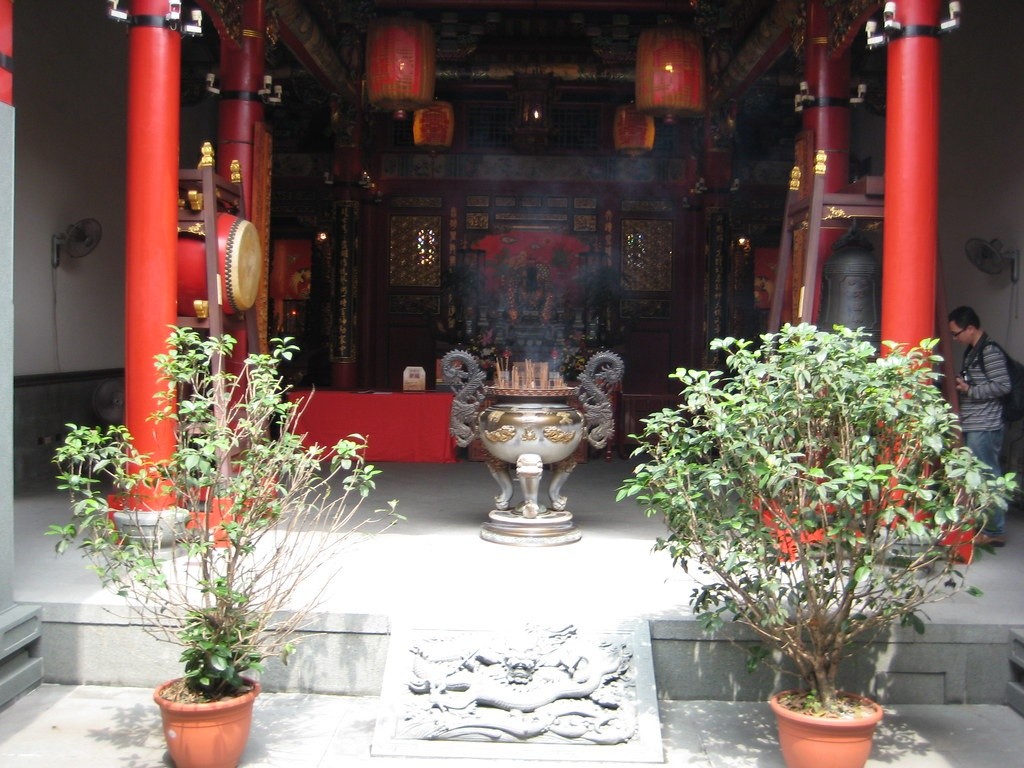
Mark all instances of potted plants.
[616,323,1018,768]
[43,325,408,767]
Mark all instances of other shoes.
[974,533,1007,546]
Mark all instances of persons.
[948,306,1011,547]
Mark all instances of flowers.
[465,328,512,379]
[548,331,598,381]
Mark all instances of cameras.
[956,370,968,384]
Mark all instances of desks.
[285,390,457,462]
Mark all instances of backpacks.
[978,341,1024,422]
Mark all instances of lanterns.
[413,101,454,153]
[365,13,436,121]
[636,16,706,126]
[613,104,655,162]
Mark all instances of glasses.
[951,329,966,336]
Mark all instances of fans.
[52,218,102,266]
[964,236,1019,282]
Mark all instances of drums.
[176,210,263,317]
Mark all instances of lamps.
[504,60,566,156]
[268,85,282,103]
[205,73,220,94]
[865,20,884,46]
[323,170,384,203]
[883,2,900,30]
[800,80,815,101]
[848,84,867,103]
[165,0,181,21]
[682,177,740,207]
[108,0,126,19]
[941,1,962,32]
[258,75,272,95]
[795,94,803,113]
[184,10,203,34]
